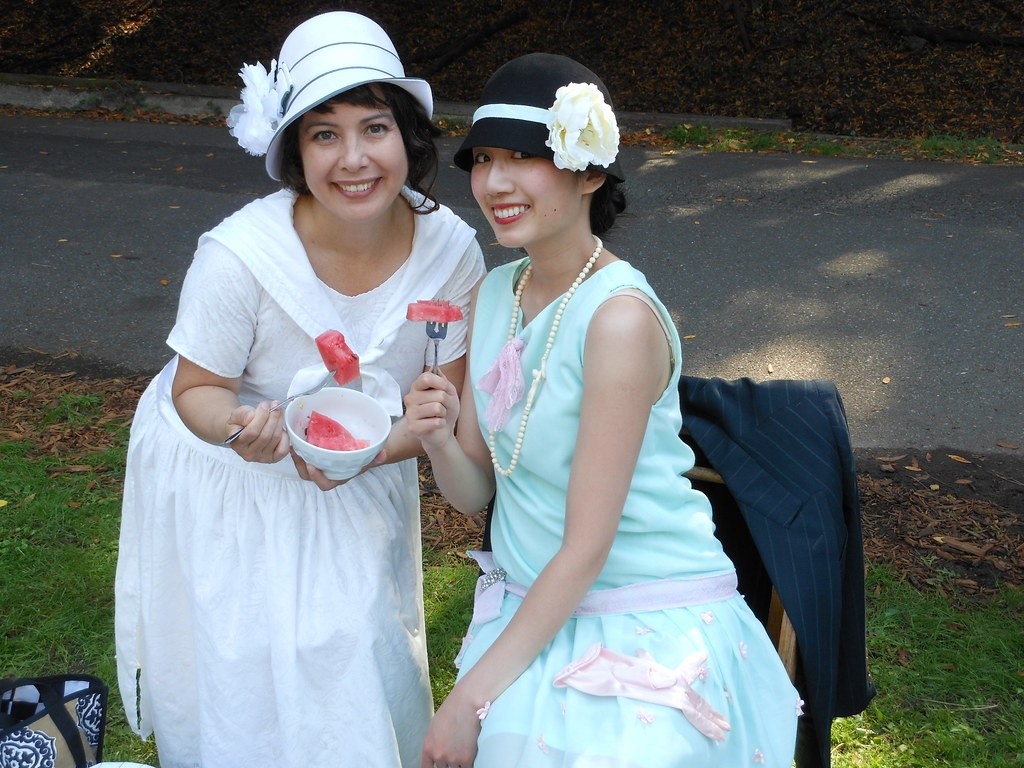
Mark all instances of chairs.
[475,376,875,768]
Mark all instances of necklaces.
[489,233,604,475]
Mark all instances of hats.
[453,52,626,187]
[225,11,433,184]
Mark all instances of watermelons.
[307,410,359,451]
[315,329,360,386]
[406,299,464,322]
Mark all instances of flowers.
[224,58,283,158]
[546,80,621,172]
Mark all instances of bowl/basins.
[285,387,392,480]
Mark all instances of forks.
[426,298,450,376]
[223,370,337,444]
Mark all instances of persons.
[111,12,486,768]
[405,54,802,768]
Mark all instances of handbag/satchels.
[0,674,109,768]
